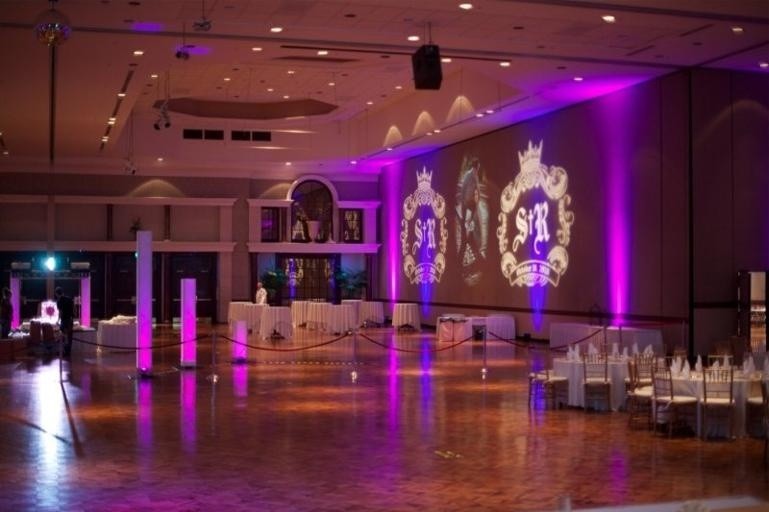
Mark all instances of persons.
[255,282,268,304]
[54,287,75,347]
[0,287,14,338]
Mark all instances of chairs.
[24,321,41,347]
[436,313,465,340]
[528,344,769,442]
[41,323,63,348]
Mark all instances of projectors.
[30,256,71,272]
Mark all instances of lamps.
[31,1,72,46]
[181,276,196,368]
[136,230,152,372]
[81,273,92,330]
[152,73,171,129]
[191,1,213,31]
[9,273,20,330]
[174,25,196,61]
[233,320,248,362]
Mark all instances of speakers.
[412,44,442,90]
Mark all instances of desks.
[466,316,516,340]
[228,300,423,342]
[98,315,137,348]
[550,321,664,359]
